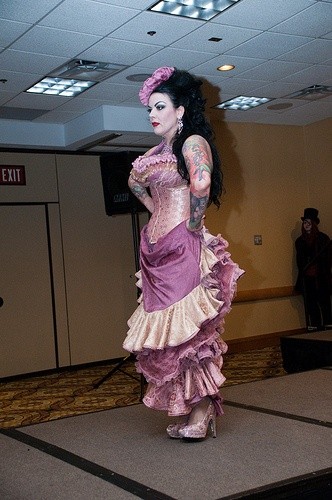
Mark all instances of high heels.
[179,402,217,441]
[166,416,189,439]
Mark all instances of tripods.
[92,214,149,398]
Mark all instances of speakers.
[99,150,151,217]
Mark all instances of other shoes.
[308,326,317,330]
[327,323,332,327]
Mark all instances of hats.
[301,208,320,224]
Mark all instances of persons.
[123,66,245,439]
[294,208,332,327]
